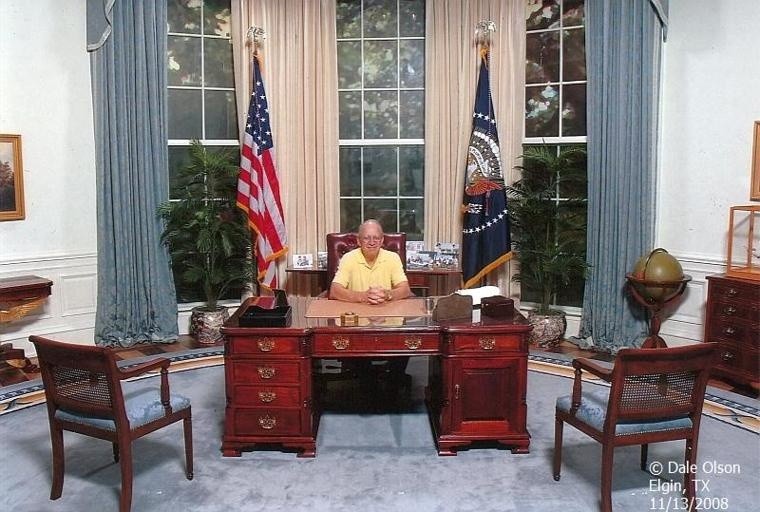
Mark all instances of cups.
[423,296,437,313]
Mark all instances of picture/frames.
[1,134,27,220]
[750,120,760,201]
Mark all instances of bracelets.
[387,290,393,302]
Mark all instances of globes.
[624,248,692,349]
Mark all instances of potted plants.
[153,136,259,345]
[505,138,588,351]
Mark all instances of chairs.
[324,229,407,387]
[553,340,721,511]
[26,334,197,512]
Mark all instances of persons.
[327,219,411,406]
[294,239,458,269]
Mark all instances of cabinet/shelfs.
[726,205,760,284]
[703,269,760,391]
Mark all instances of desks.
[0,276,53,374]
[218,297,532,458]
[287,261,465,297]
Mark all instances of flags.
[462,49,512,291]
[236,51,289,292]
